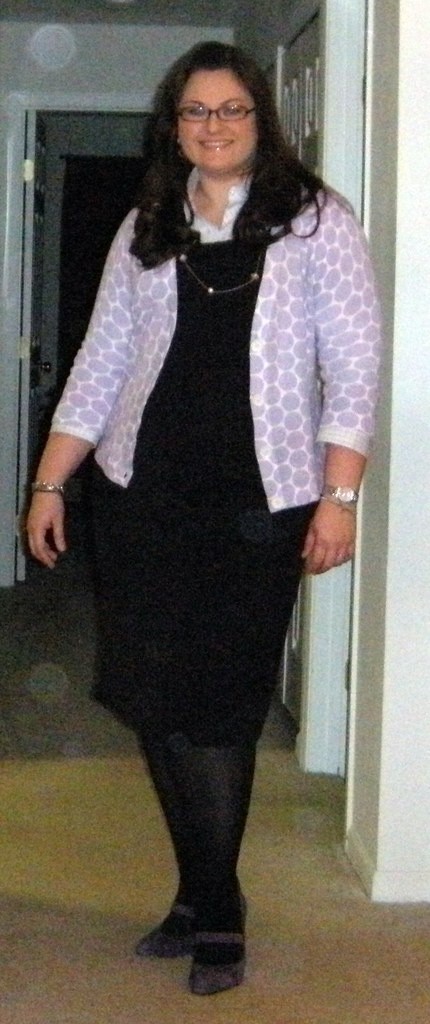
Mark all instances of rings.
[337,553,346,557]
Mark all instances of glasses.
[173,104,258,122]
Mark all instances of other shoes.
[189,894,248,996]
[134,900,197,957]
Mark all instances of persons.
[26,43,382,994]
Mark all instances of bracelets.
[321,496,355,515]
[31,482,65,494]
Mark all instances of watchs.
[322,485,359,502]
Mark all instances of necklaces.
[179,248,262,294]
